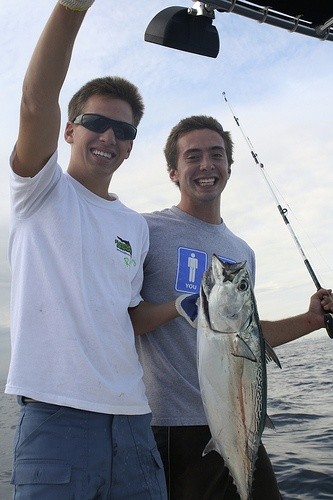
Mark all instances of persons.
[134,115,333,500]
[4,0,199,500]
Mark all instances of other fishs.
[195,252,283,500]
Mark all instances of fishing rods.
[221,90,333,338]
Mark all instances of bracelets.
[58,0,95,11]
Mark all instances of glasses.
[73,113,137,141]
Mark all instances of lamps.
[144,0,220,58]
